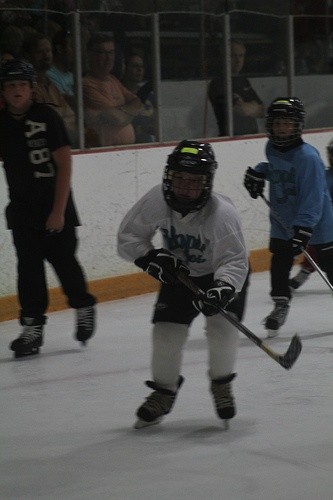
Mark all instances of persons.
[117,140,251,423]
[243,97,333,331]
[208,33,265,137]
[0,58,97,353]
[158,0,333,81]
[0,0,158,149]
[287,140,333,292]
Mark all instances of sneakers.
[289,267,312,293]
[132,391,176,430]
[210,380,237,430]
[262,301,291,338]
[74,305,96,347]
[9,324,44,359]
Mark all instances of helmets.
[0,58,36,81]
[265,97,307,152]
[162,140,218,213]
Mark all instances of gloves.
[244,166,267,198]
[288,226,314,256]
[192,280,237,317]
[137,248,190,285]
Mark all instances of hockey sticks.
[261,192,333,290]
[177,272,304,374]
